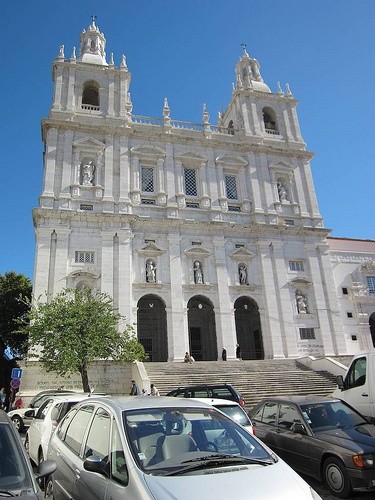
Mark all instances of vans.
[331,348,375,421]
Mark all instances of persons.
[146,261,157,283]
[130,380,140,396]
[189,356,195,364]
[185,352,190,363]
[222,347,226,361]
[0,387,22,413]
[236,344,242,361]
[148,384,160,396]
[81,160,95,186]
[141,389,148,396]
[278,181,289,201]
[194,261,204,284]
[297,290,308,314]
[239,265,248,285]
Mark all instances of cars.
[23,394,110,475]
[162,397,256,457]
[43,395,323,499]
[6,390,84,434]
[247,394,375,498]
[0,409,58,500]
[165,383,246,408]
